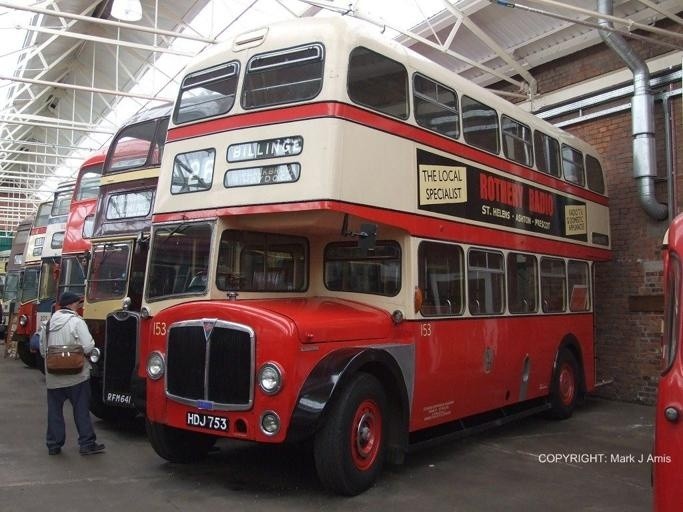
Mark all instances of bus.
[651,212,683,512]
[0,17,614,496]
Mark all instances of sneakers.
[48,448,61,455]
[80,442,105,454]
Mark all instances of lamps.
[110,0,143,22]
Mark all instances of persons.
[37,291,108,455]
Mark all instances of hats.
[60,292,81,307]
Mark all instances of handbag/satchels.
[46,344,84,375]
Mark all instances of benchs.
[423,297,551,317]
